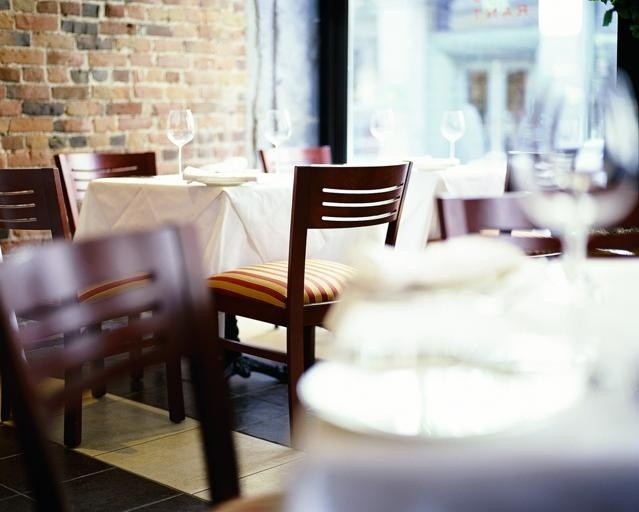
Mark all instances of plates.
[297,358,592,440]
[185,172,257,187]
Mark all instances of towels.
[184,154,248,185]
[329,231,586,384]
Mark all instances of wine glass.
[167,108,196,182]
[439,109,466,165]
[510,67,635,283]
[371,106,399,165]
[262,109,291,173]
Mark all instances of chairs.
[0,167,186,447]
[0,223,286,512]
[203,161,412,448]
[500,148,578,237]
[436,187,639,259]
[259,145,332,173]
[53,151,156,238]
